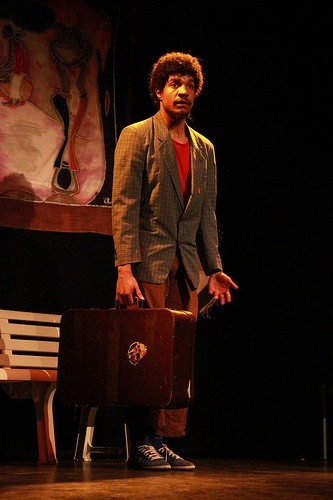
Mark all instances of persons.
[111,53,241,472]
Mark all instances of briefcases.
[57,296,196,409]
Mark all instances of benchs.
[0,309,130,464]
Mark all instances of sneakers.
[158,444,195,470]
[128,442,172,470]
